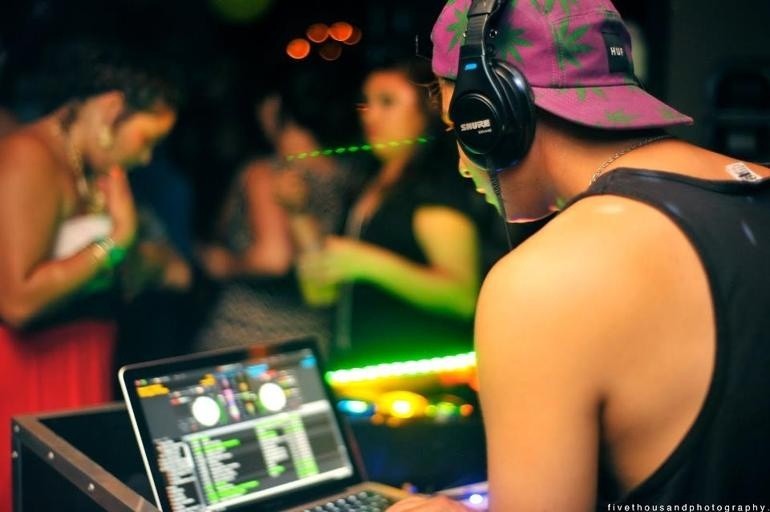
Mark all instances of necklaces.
[588,134,672,188]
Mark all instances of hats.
[430,0,695,131]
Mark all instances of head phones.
[447,0,540,175]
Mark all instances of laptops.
[117,333,414,512]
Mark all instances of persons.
[1,41,486,511]
[383,1,768,511]
